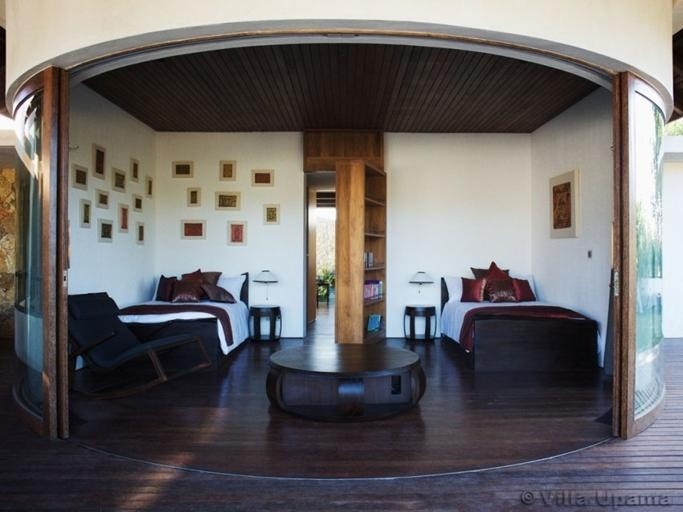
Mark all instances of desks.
[404,306,437,344]
[247,306,283,346]
[264,340,428,424]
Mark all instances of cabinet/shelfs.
[334,157,388,345]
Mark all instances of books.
[367,314,382,331]
[364,279,382,301]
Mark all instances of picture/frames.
[262,203,280,224]
[217,159,236,182]
[186,187,201,207]
[214,191,241,211]
[170,159,194,178]
[547,168,581,239]
[227,219,247,246]
[181,218,205,240]
[72,142,155,246]
[251,167,274,187]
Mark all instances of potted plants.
[316,266,336,307]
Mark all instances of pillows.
[155,268,235,303]
[460,262,535,302]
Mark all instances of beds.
[119,266,250,375]
[441,275,598,390]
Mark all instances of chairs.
[67,291,212,403]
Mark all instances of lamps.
[409,271,435,304]
[253,267,279,306]
